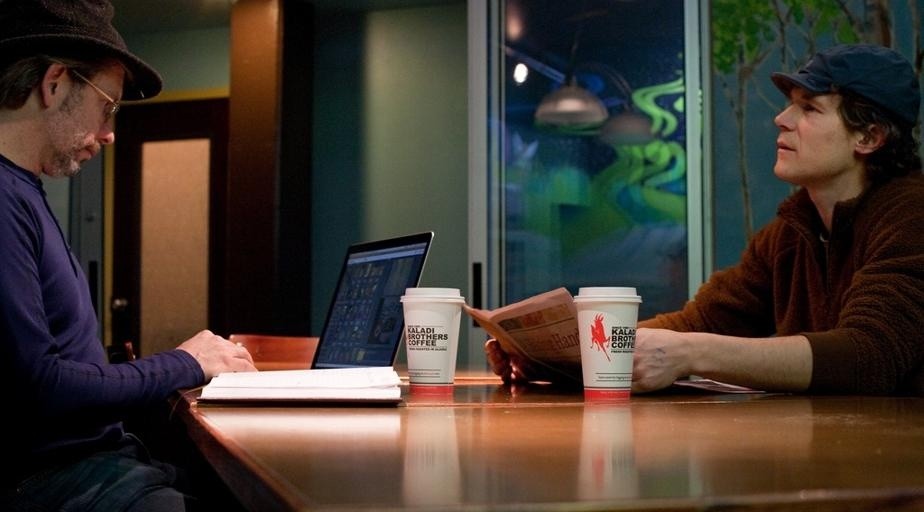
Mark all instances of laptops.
[311,231,435,370]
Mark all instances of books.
[463,287,584,387]
[194,362,404,405]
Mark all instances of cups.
[399,286,465,398]
[572,285,642,406]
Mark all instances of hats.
[771,43,921,128]
[0,0,163,102]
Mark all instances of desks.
[169,364,912,512]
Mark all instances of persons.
[0,1,262,511]
[482,42,923,398]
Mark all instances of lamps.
[534,61,653,147]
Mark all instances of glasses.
[45,55,120,114]
